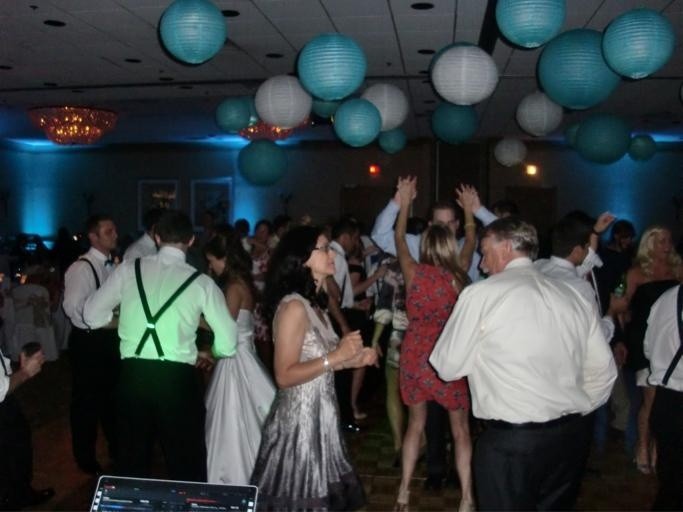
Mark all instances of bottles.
[613,282,626,297]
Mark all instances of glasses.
[314,245,330,252]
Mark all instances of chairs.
[30,273,44,284]
[48,280,64,301]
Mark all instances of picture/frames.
[137,179,182,231]
[190,177,233,227]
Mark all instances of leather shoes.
[35,488,54,503]
[90,464,103,477]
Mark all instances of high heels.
[631,438,657,475]
[393,492,410,512]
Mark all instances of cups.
[21,340,46,380]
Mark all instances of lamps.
[27,107,118,144]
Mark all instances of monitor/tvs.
[88,474,260,512]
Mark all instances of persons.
[1,174,683,512]
